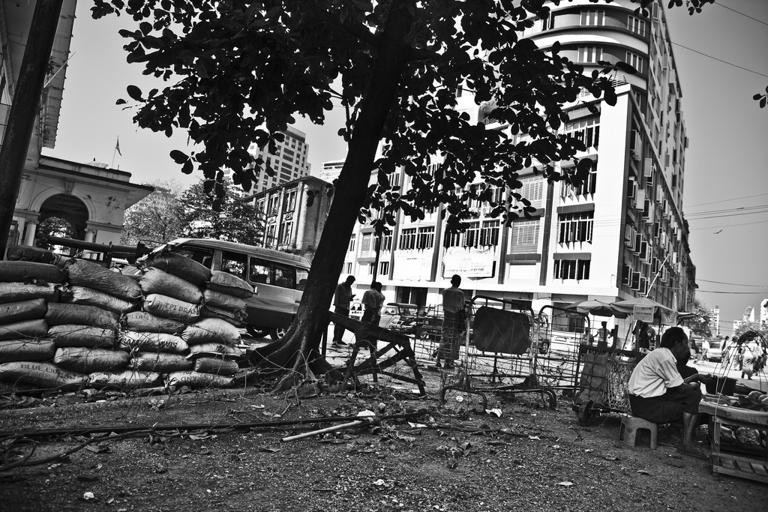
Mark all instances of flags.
[115,136,125,157]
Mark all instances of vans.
[119,237,311,340]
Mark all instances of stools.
[620,413,656,448]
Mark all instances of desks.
[697,393,767,486]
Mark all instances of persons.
[593,321,610,348]
[360,282,384,350]
[582,327,594,346]
[331,275,355,346]
[631,321,652,353]
[607,324,618,348]
[626,327,699,448]
[434,274,466,369]
[700,333,768,379]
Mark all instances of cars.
[706,341,722,361]
[381,295,551,356]
